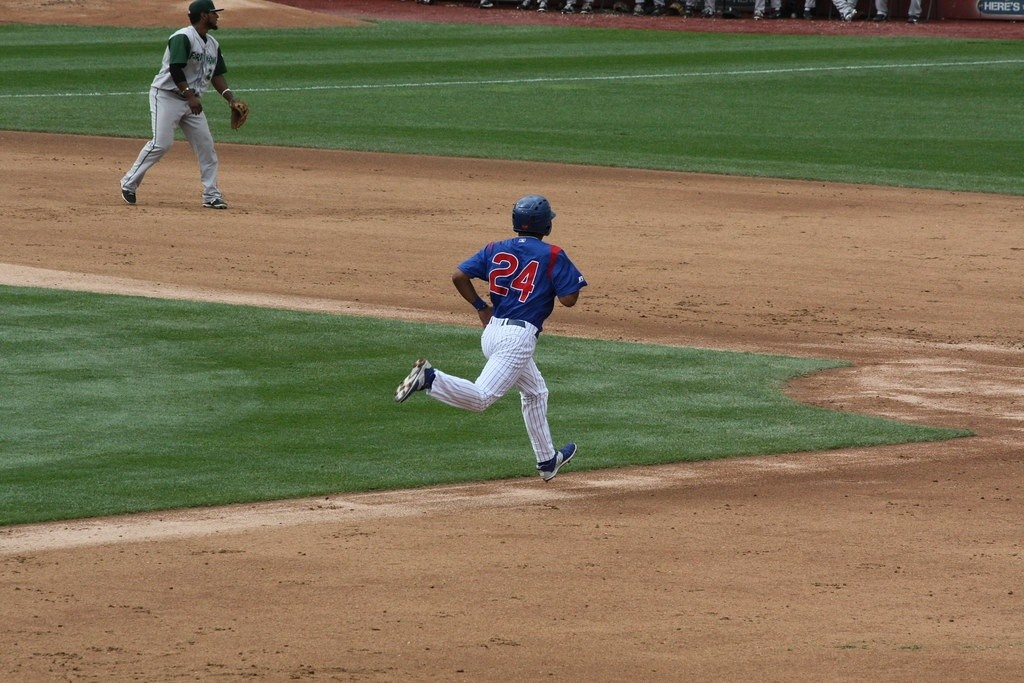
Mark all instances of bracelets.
[222,88,232,96]
[181,86,189,95]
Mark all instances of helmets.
[512,194,557,233]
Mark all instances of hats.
[188,0,224,15]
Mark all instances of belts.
[491,319,540,339]
[172,90,187,98]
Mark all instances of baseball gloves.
[230,100,249,130]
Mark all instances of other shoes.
[873,14,885,21]
[686,7,696,17]
[581,2,593,14]
[652,6,665,16]
[633,4,645,16]
[907,14,920,23]
[769,11,783,19]
[613,3,628,12]
[804,10,812,20]
[754,10,763,20]
[722,6,738,19]
[479,0,495,8]
[516,0,531,10]
[561,4,576,14]
[842,9,857,22]
[536,0,548,12]
[702,8,715,18]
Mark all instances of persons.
[425,0,923,25]
[394,193,590,482]
[119,0,237,208]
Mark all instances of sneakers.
[394,358,435,403]
[536,443,577,481]
[203,197,228,209]
[121,190,136,204]
